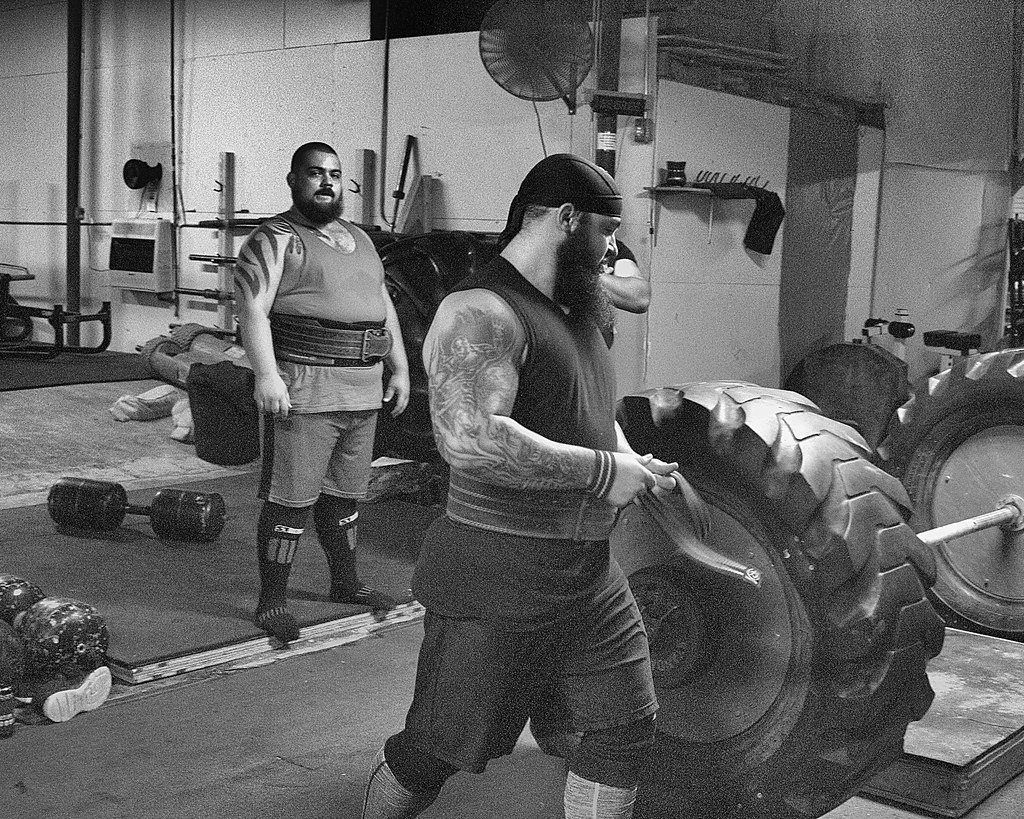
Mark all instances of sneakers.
[31,663,112,724]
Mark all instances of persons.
[232,141,411,647]
[359,150,681,819]
[599,235,654,314]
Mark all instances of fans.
[477,0,596,115]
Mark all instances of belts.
[269,314,393,368]
[445,467,617,541]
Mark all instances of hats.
[495,154,623,244]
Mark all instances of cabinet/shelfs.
[173,145,374,338]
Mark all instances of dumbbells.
[48,476,226,543]
